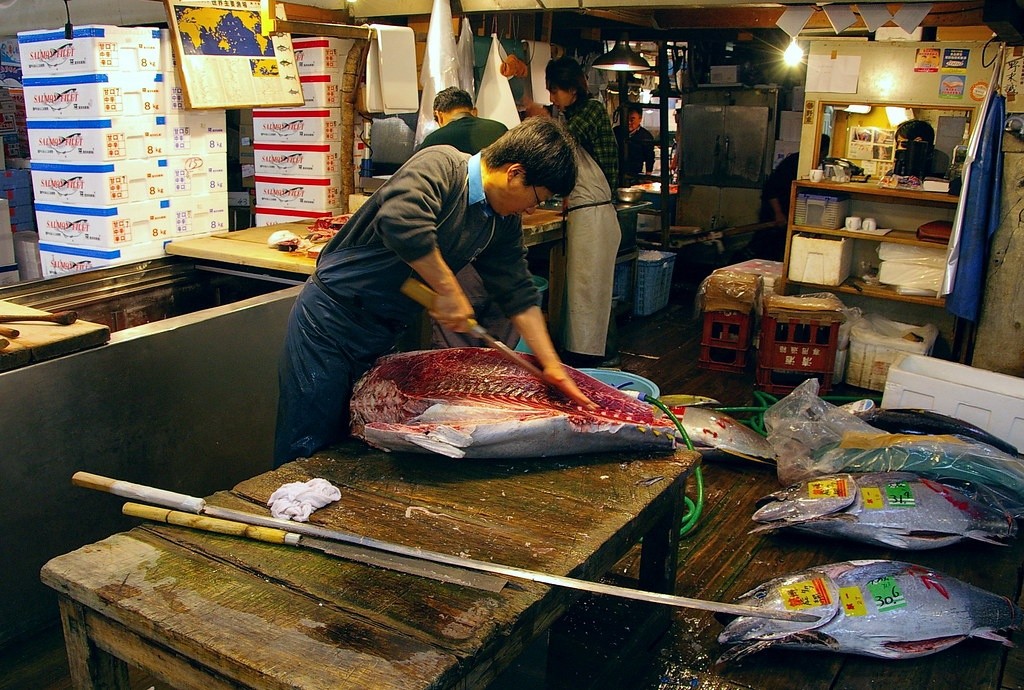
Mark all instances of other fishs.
[658,404,778,466]
[710,558,1023,668]
[348,346,678,459]
[746,469,1024,551]
[856,408,1024,461]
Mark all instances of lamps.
[591,27,651,71]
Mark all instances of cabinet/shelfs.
[779,178,959,308]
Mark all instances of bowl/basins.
[575,367,660,405]
[616,188,645,202]
[661,394,722,418]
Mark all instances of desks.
[44,438,703,690]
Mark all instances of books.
[922,177,950,192]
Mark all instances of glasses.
[533,185,545,209]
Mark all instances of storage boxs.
[710,66,740,83]
[614,250,677,317]
[794,192,850,230]
[773,86,805,169]
[696,232,1024,466]
[0,25,372,285]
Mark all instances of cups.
[862,217,877,232]
[845,216,861,230]
[809,170,823,183]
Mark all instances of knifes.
[120,501,507,595]
[402,278,569,396]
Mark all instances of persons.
[613,104,655,178]
[895,120,950,180]
[272,114,576,473]
[546,55,619,196]
[745,134,831,250]
[563,144,622,368]
[419,87,509,155]
